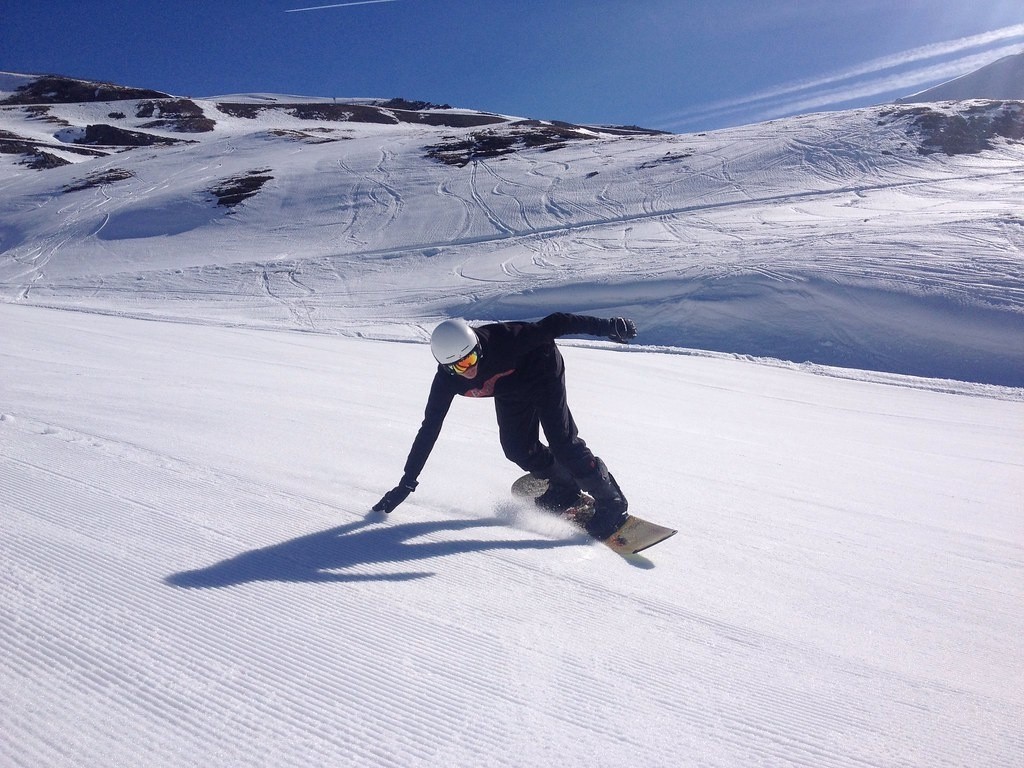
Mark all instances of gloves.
[372,476,419,514]
[596,317,637,343]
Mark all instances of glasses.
[441,343,483,376]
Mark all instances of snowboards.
[511,473,679,554]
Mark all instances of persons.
[371,312,636,540]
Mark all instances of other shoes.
[534,478,581,512]
[585,495,629,541]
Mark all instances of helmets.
[430,320,483,375]
